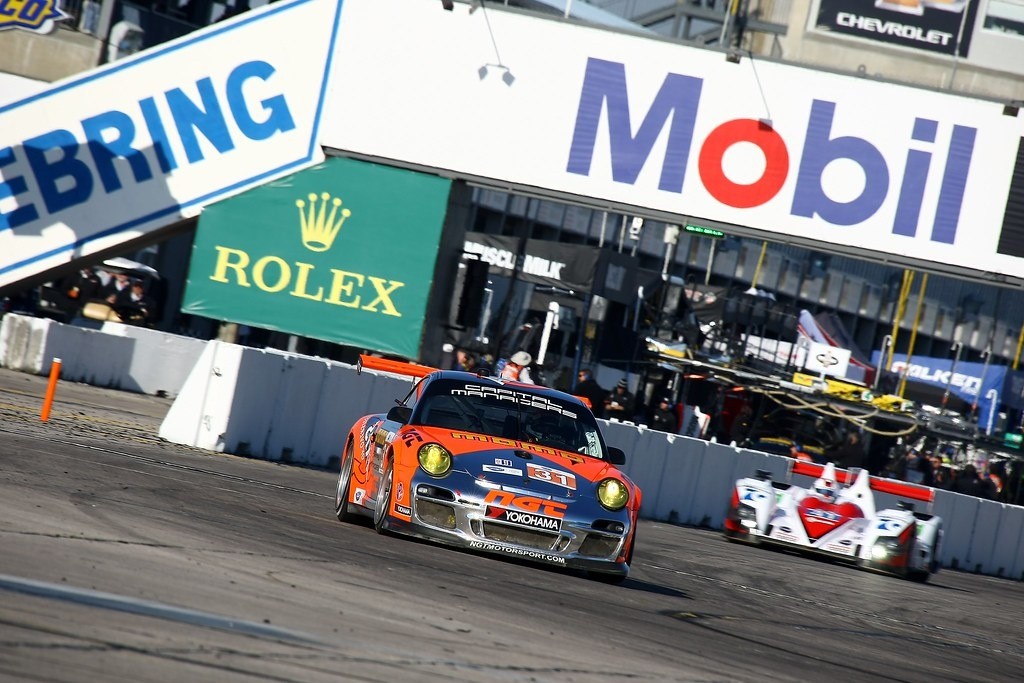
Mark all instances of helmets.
[525,413,560,440]
[812,478,838,501]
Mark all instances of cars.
[335,368,643,585]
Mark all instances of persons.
[434,340,1024,509]
[31,265,161,338]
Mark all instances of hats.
[617,379,627,389]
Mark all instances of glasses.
[580,375,585,377]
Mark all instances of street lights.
[940,341,963,412]
[968,349,992,422]
[985,389,998,436]
[873,335,892,389]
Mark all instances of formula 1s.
[720,462,945,583]
